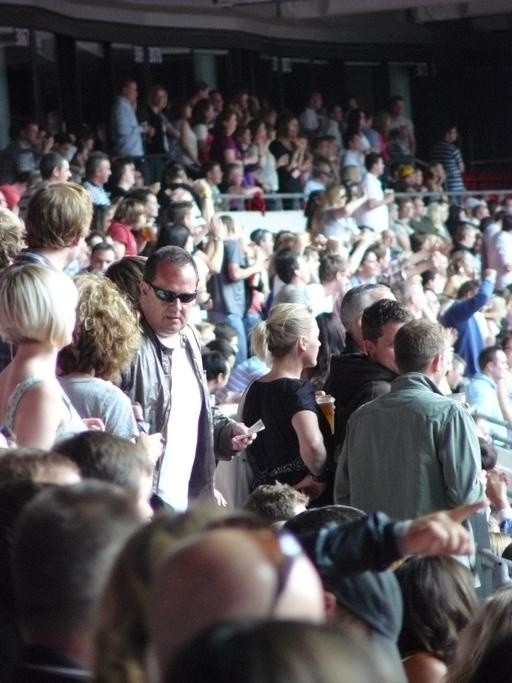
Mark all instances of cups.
[143,222,156,242]
[314,393,336,436]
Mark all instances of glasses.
[143,277,199,304]
[201,508,308,621]
